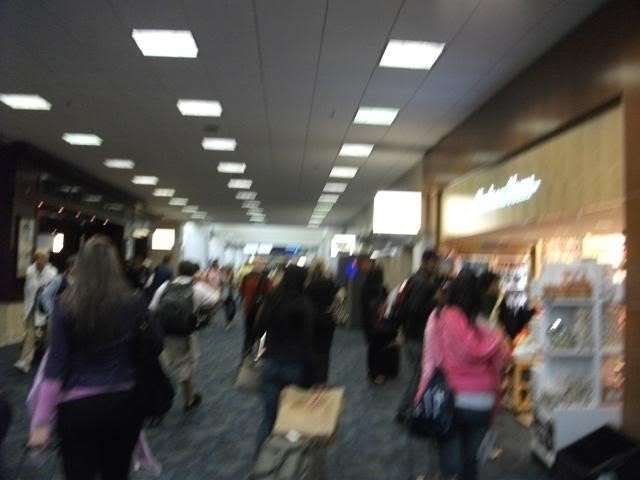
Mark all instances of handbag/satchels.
[236,332,268,395]
[395,360,458,441]
[273,382,345,446]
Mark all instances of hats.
[177,259,200,277]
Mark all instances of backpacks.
[154,279,206,337]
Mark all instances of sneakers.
[14,361,30,374]
[185,392,202,410]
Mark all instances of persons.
[12,232,540,480]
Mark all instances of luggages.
[244,436,329,479]
